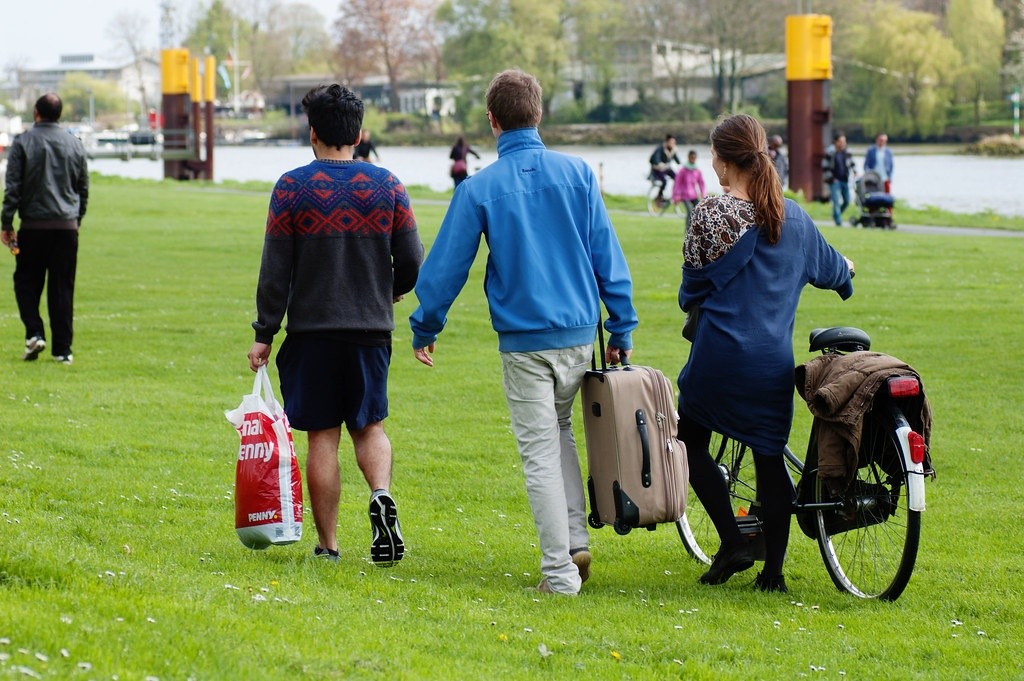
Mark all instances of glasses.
[486,111,498,122]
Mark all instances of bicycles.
[647,171,689,219]
[675,269,937,605]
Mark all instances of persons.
[447,135,481,190]
[353,129,381,168]
[410,69,638,598]
[676,112,856,596]
[248,85,425,570]
[2,94,88,363]
[647,130,894,232]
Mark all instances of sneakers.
[697,537,754,587]
[52,354,73,366]
[745,571,788,595]
[368,487,404,568]
[24,334,46,361]
[309,547,341,566]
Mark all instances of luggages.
[580,306,689,536]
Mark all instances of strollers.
[851,170,895,232]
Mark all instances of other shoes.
[571,548,591,583]
[535,578,552,594]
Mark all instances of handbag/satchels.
[453,159,466,174]
[224,364,303,550]
[682,304,699,343]
